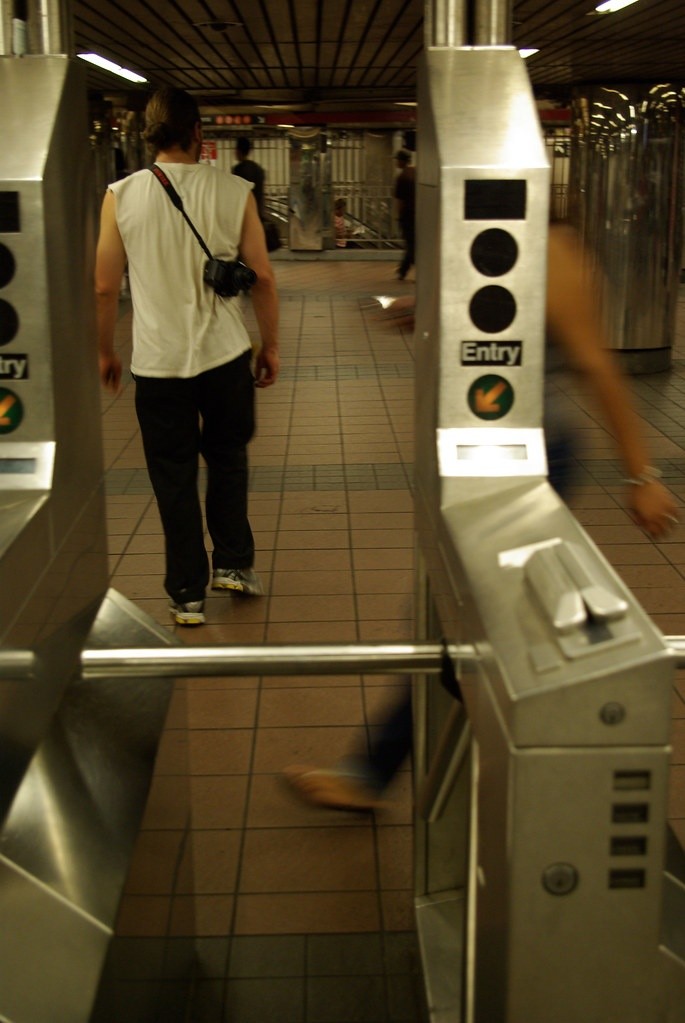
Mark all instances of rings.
[671,518,678,525]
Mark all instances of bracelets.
[627,469,662,487]
[99,341,117,356]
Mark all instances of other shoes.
[169,592,205,625]
[213,564,264,595]
[284,764,382,812]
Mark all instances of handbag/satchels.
[261,219,284,253]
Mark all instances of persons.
[334,199,355,248]
[279,212,678,818]
[390,149,414,280]
[96,91,279,626]
[230,135,263,213]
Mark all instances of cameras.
[203,260,257,298]
